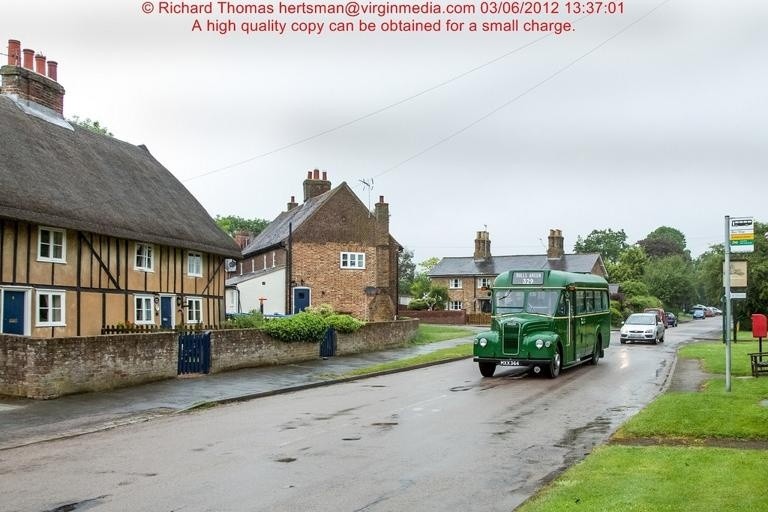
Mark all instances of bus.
[474,268,611,378]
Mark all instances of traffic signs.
[730,217,754,253]
[723,291,747,300]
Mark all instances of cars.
[690,304,721,318]
[620,307,677,343]
